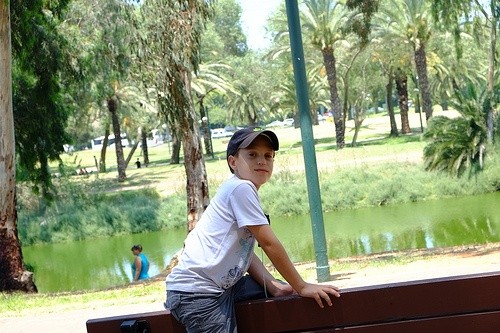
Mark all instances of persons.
[166,128,341,333]
[134,158,141,168]
[93,156,99,170]
[78,167,88,175]
[131,244,150,282]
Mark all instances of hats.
[225,127,280,156]
